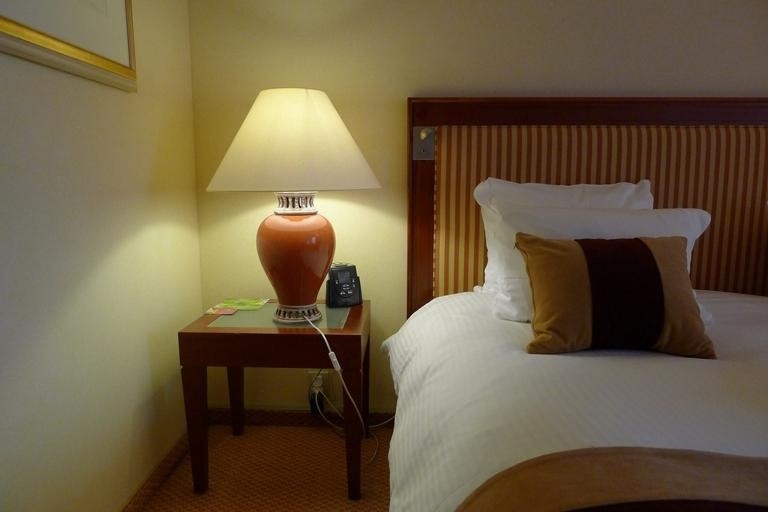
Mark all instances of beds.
[391,94,767,512]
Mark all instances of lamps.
[205,86,382,328]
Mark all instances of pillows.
[513,231,718,360]
[471,177,654,298]
[490,196,712,322]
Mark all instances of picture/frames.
[0,0,142,95]
